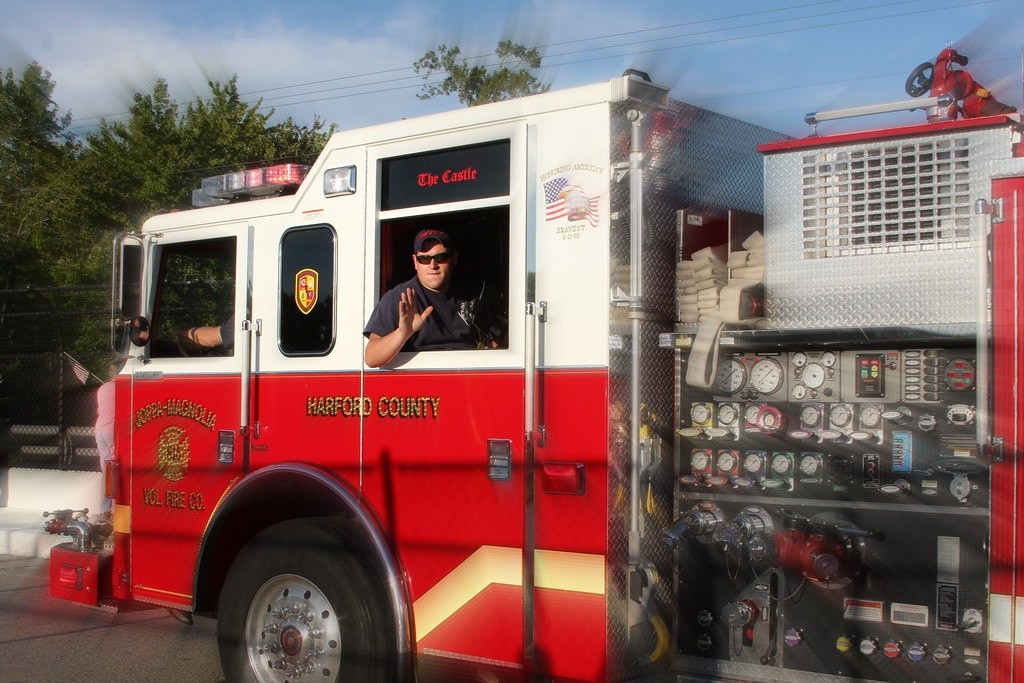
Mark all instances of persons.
[175,312,235,347]
[362,227,502,368]
[94,359,117,515]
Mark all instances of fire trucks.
[39,43,1024,683]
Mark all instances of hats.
[413,228,453,253]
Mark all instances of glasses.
[415,252,452,265]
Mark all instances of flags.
[66,355,90,387]
